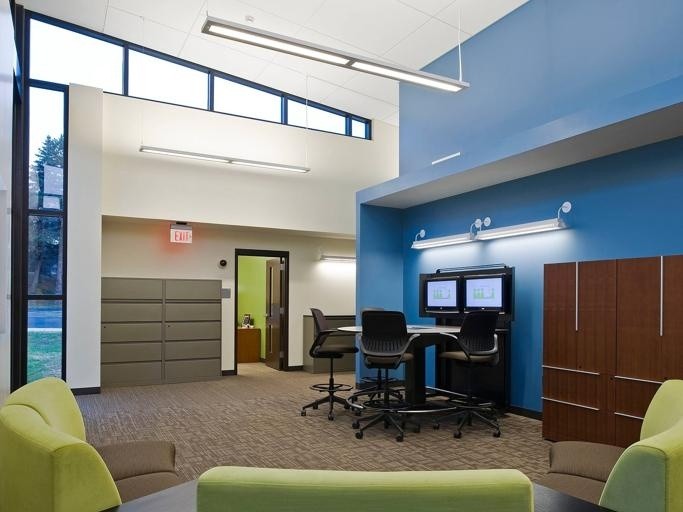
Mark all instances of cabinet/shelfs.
[101,276,222,388]
[542,258,617,446]
[617,255,683,448]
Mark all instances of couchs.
[0,377,182,512]
[116,466,619,512]
[532,379,683,512]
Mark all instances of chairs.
[300,308,359,420]
[350,307,421,442]
[431,310,510,438]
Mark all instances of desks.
[303,315,356,373]
[336,324,510,412]
[238,328,261,363]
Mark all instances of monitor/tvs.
[425,276,460,314]
[464,274,505,314]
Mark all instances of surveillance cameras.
[218,259,228,268]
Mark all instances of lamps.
[320,255,356,263]
[411,218,481,249]
[137,16,311,174]
[201,0,470,95]
[476,202,571,240]
